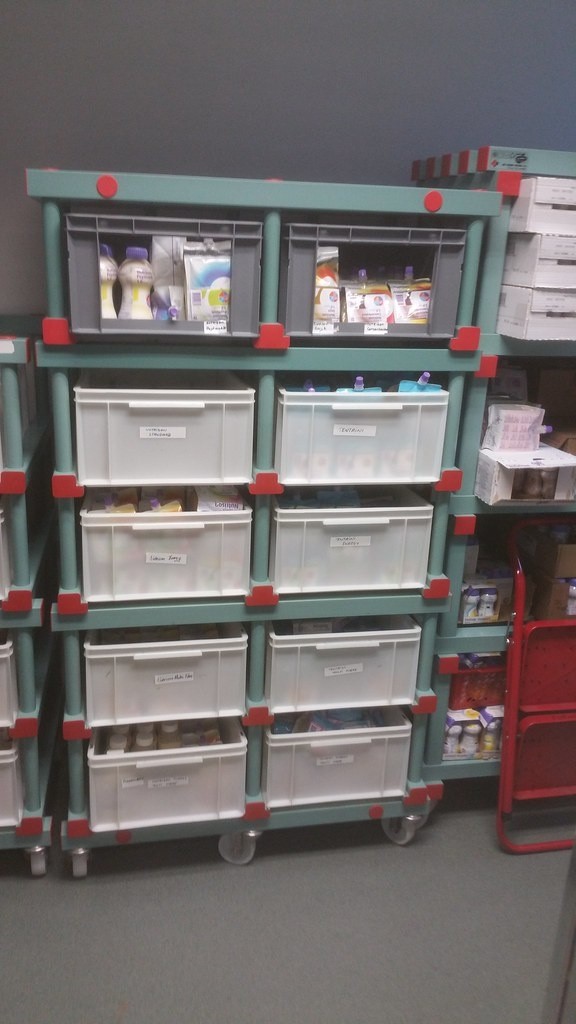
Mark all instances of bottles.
[444,717,503,752]
[118,247,154,320]
[478,589,497,617]
[463,589,480,618]
[566,579,576,615]
[99,243,118,320]
[102,720,182,754]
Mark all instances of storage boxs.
[84,622,247,728]
[496,175,575,343]
[265,616,422,714]
[80,490,253,602]
[272,489,435,595]
[444,668,505,759]
[279,223,468,338]
[73,370,255,486]
[264,707,413,809]
[64,214,264,336]
[457,524,576,625]
[87,717,249,832]
[271,381,450,486]
[475,441,576,505]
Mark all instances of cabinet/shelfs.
[410,145,576,827]
[0,313,59,874]
[25,168,501,877]
